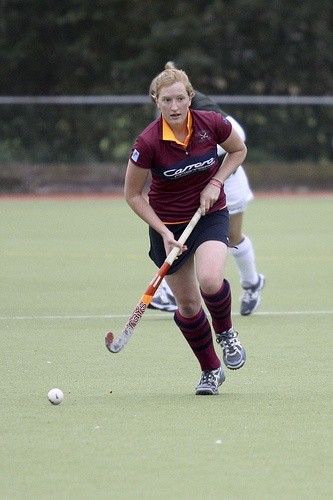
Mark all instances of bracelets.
[211,177,223,186]
[210,180,221,188]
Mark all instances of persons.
[147,89,265,316]
[124,61,247,396]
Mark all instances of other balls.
[46,386,64,405]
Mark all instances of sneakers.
[147,288,178,312]
[196,366,225,395]
[240,274,265,315]
[216,326,246,370]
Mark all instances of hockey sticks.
[105,203,204,354]
